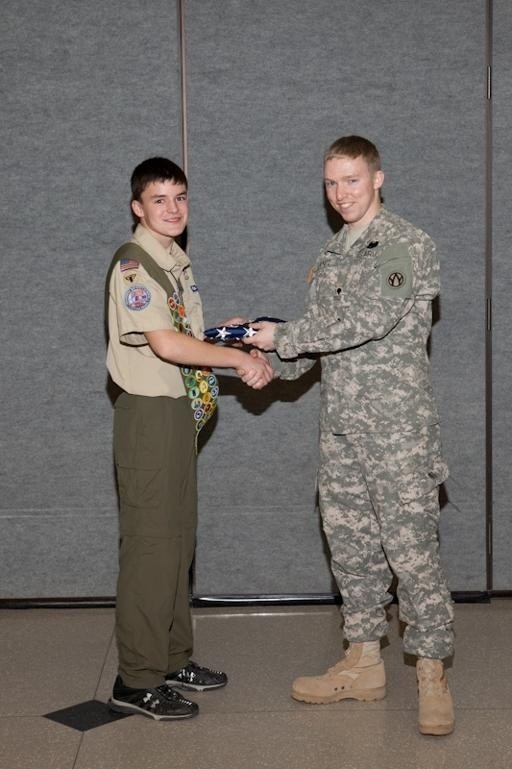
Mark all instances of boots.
[290,638,387,705]
[416,656,455,736]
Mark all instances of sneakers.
[165,662,227,692]
[108,675,199,721]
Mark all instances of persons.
[100,154,275,723]
[233,134,458,737]
